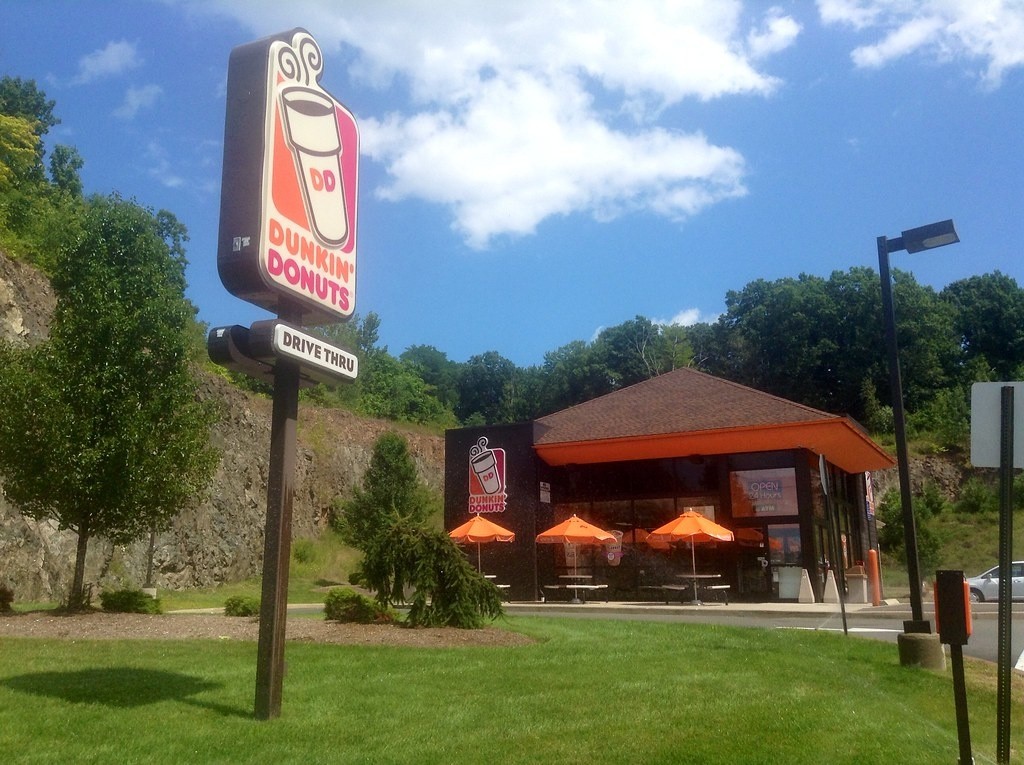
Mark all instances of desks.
[484,574,497,579]
[559,574,592,604]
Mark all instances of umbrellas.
[534,514,617,603]
[447,514,516,571]
[646,508,735,603]
[624,527,780,551]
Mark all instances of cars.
[966,560,1024,602]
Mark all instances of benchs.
[661,584,731,605]
[496,585,511,604]
[544,585,608,604]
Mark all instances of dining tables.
[675,574,722,605]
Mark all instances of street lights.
[876,218,960,669]
